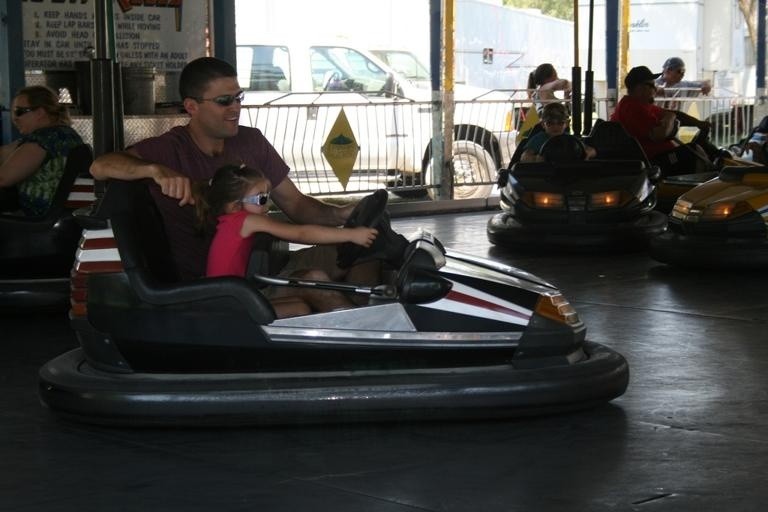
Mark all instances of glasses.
[12,105,33,116]
[202,88,243,106]
[241,191,272,206]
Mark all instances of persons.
[654,58,715,113]
[190,163,379,320]
[520,103,598,163]
[89,56,382,312]
[527,64,573,133]
[609,66,724,185]
[0,85,84,219]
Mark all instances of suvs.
[368,43,513,106]
[233,32,519,203]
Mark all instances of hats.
[543,102,569,123]
[624,66,663,86]
